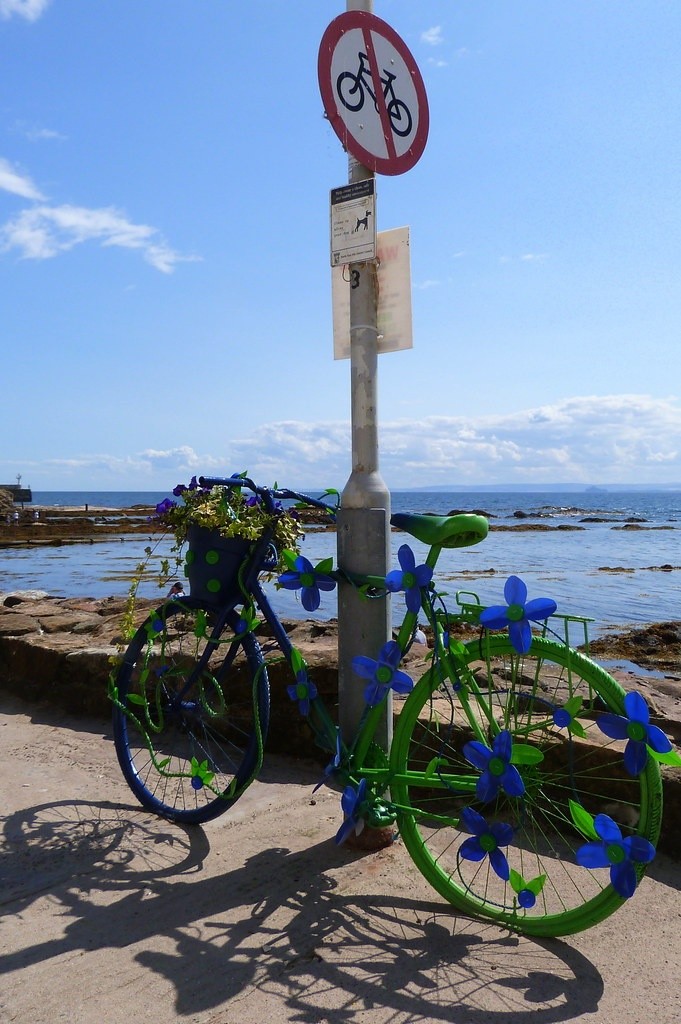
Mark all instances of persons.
[34,509,40,524]
[13,510,19,527]
[5,513,12,528]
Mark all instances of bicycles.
[111,475,666,938]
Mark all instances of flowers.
[103,472,680,910]
